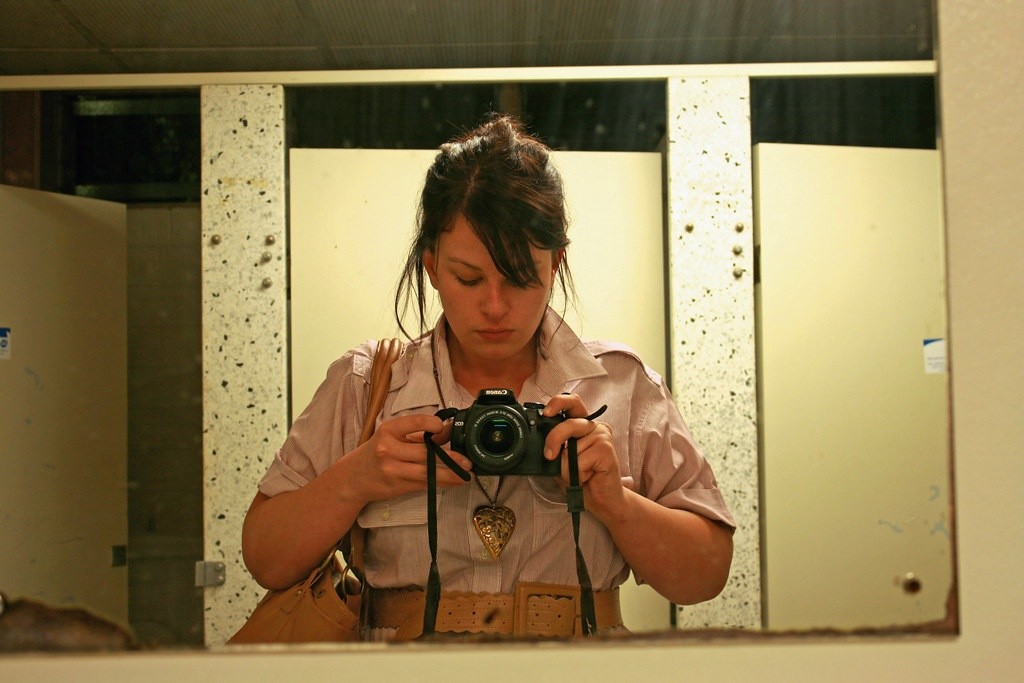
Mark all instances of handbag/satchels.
[225,336,402,646]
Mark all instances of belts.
[364,581,624,637]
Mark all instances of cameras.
[450,388,564,476]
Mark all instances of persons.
[242,111,737,644]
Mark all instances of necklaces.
[431,332,516,562]
